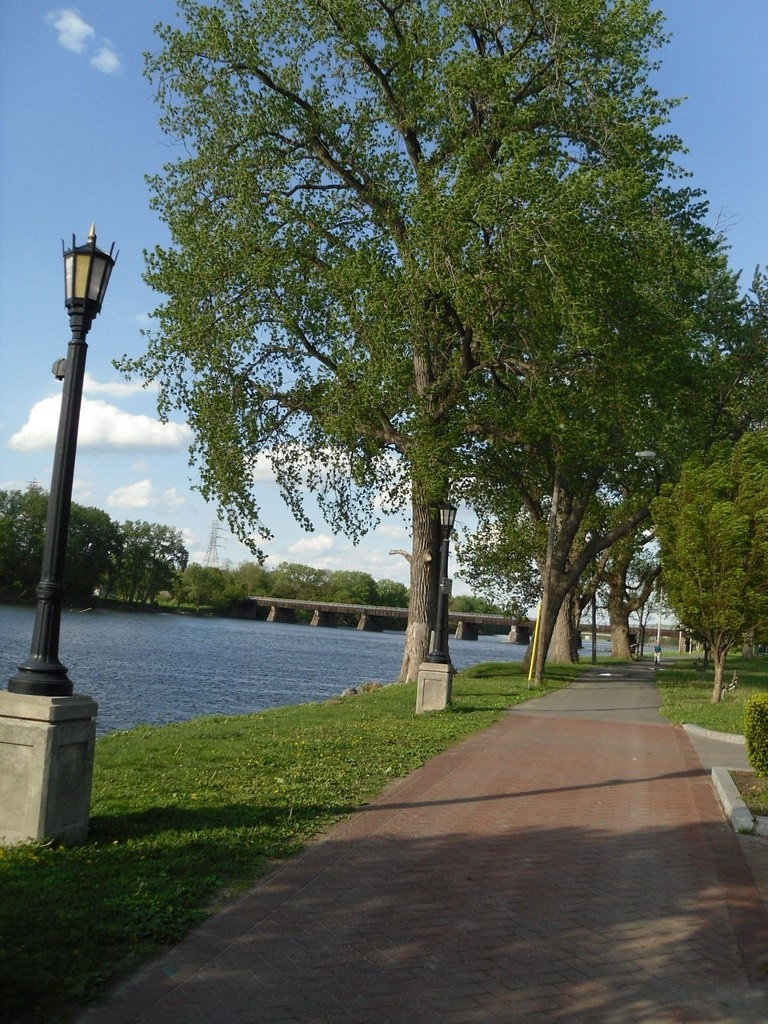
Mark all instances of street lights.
[424,500,457,663]
[5,220,122,696]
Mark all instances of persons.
[653,642,661,665]
